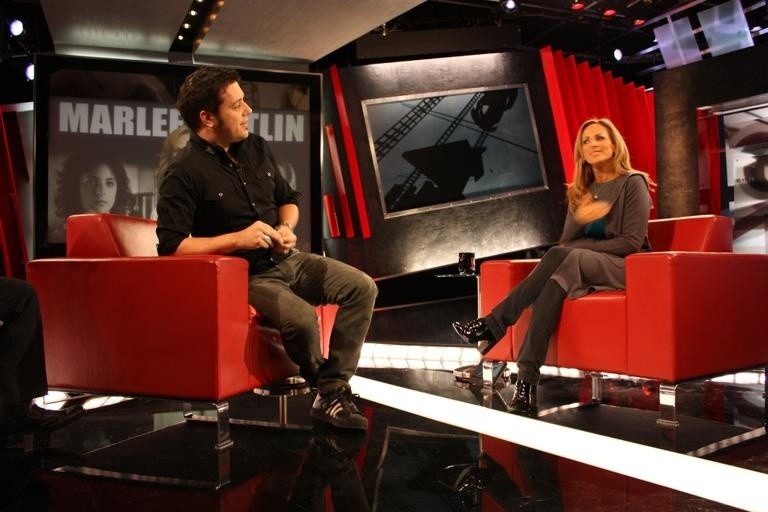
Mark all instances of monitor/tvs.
[360,82,549,219]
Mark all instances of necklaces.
[593,180,609,200]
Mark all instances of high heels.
[455,449,505,496]
[451,318,497,355]
[506,380,539,411]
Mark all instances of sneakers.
[308,388,370,431]
[310,430,367,465]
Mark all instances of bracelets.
[276,222,294,232]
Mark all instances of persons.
[0,275,85,446]
[456,424,570,511]
[451,116,659,416]
[150,126,195,199]
[150,64,381,434]
[52,144,141,228]
[279,425,377,511]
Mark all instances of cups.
[459,252,475,276]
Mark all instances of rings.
[264,234,270,241]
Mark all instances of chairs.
[480,214,767,455]
[22,212,342,494]
